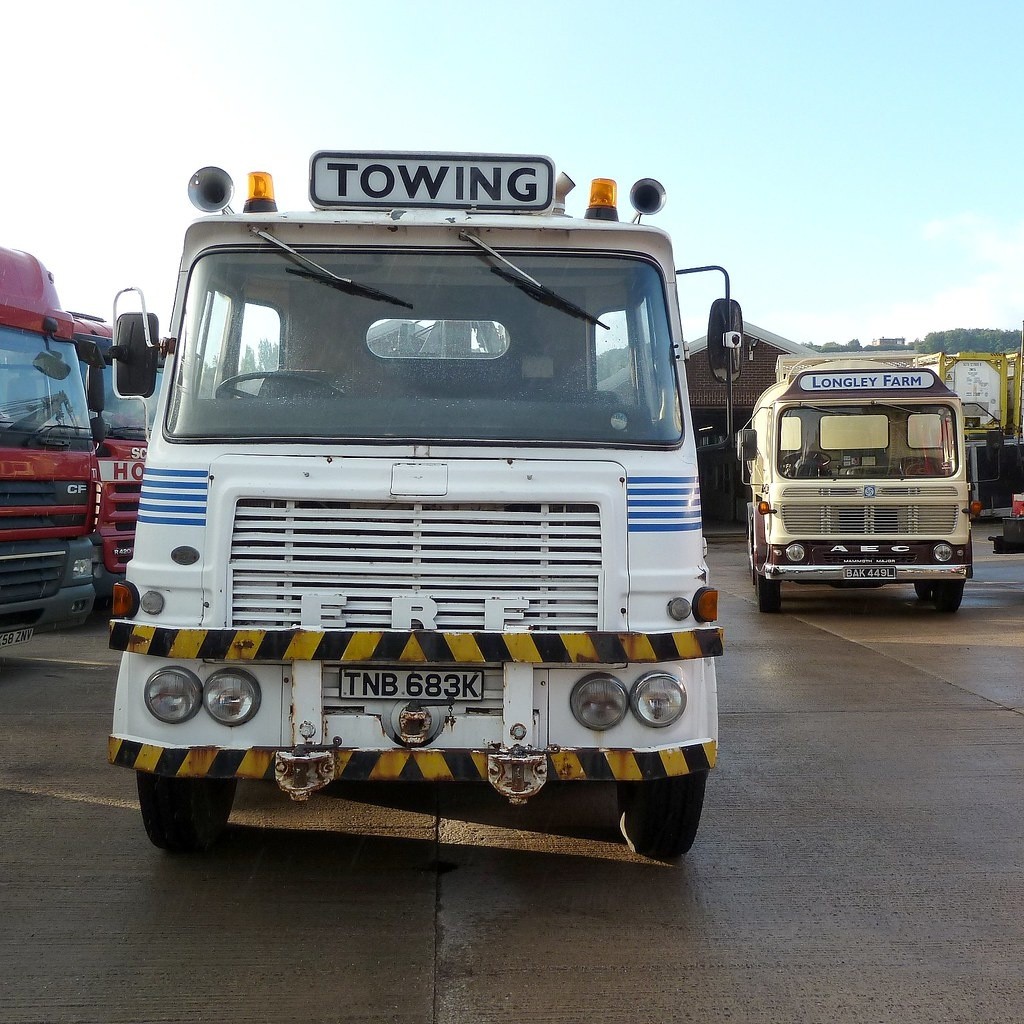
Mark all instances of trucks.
[65,311,184,642]
[1,245,109,655]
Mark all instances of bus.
[109,147,739,869]
[734,349,1007,619]
